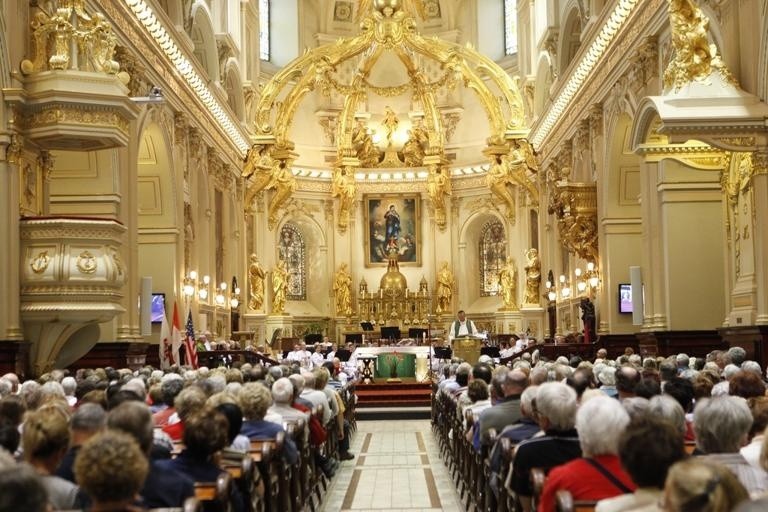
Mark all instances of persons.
[246,103,527,225]
[248,248,541,318]
[0,332,358,511]
[361,338,414,346]
[384,204,401,254]
[448,308,478,343]
[427,333,767,512]
[621,292,629,302]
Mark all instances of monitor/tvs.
[381,327,399,339]
[136,292,165,323]
[618,283,645,313]
[409,328,428,338]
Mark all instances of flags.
[158,298,170,371]
[170,300,186,368]
[183,307,201,370]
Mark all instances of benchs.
[157,436,286,512]
[556,440,697,512]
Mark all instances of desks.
[357,355,377,385]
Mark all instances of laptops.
[360,322,374,331]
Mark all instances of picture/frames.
[363,192,423,267]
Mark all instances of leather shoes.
[341,454,355,461]
[322,458,337,477]
